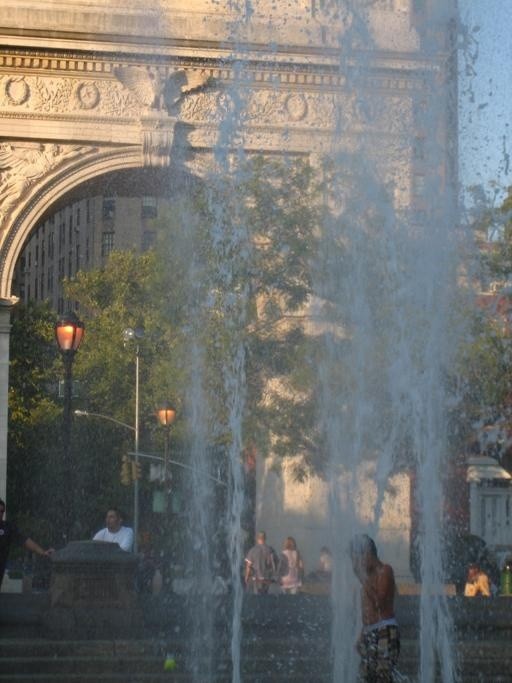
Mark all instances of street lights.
[56,309,84,546]
[151,396,177,590]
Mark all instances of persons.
[278,535,306,594]
[346,532,402,682]
[318,546,333,581]
[92,506,134,552]
[462,561,491,597]
[244,530,279,594]
[0,499,57,583]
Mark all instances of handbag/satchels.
[297,567,305,586]
[266,552,277,580]
